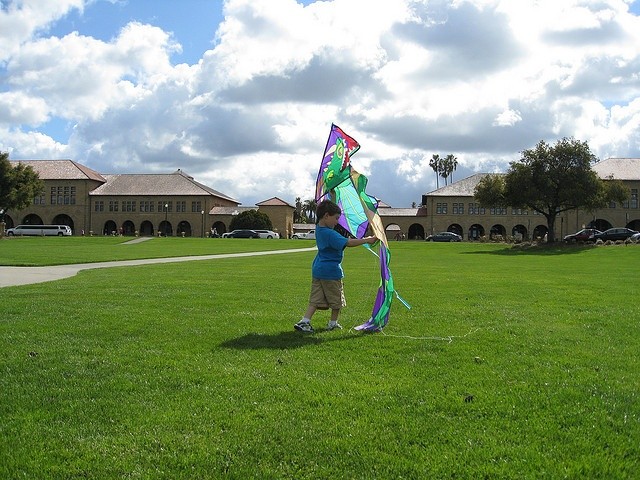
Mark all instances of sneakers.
[294,320,314,333]
[327,323,343,332]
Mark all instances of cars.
[564,229,600,243]
[631,233,640,242]
[426,232,462,242]
[589,228,638,240]
[227,230,259,239]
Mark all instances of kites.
[314,123,410,333]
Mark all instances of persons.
[213,227,218,237]
[394,233,406,241]
[483,234,486,243]
[294,200,381,331]
[209,228,213,237]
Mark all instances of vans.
[222,229,279,239]
[291,230,316,240]
[6,225,72,237]
